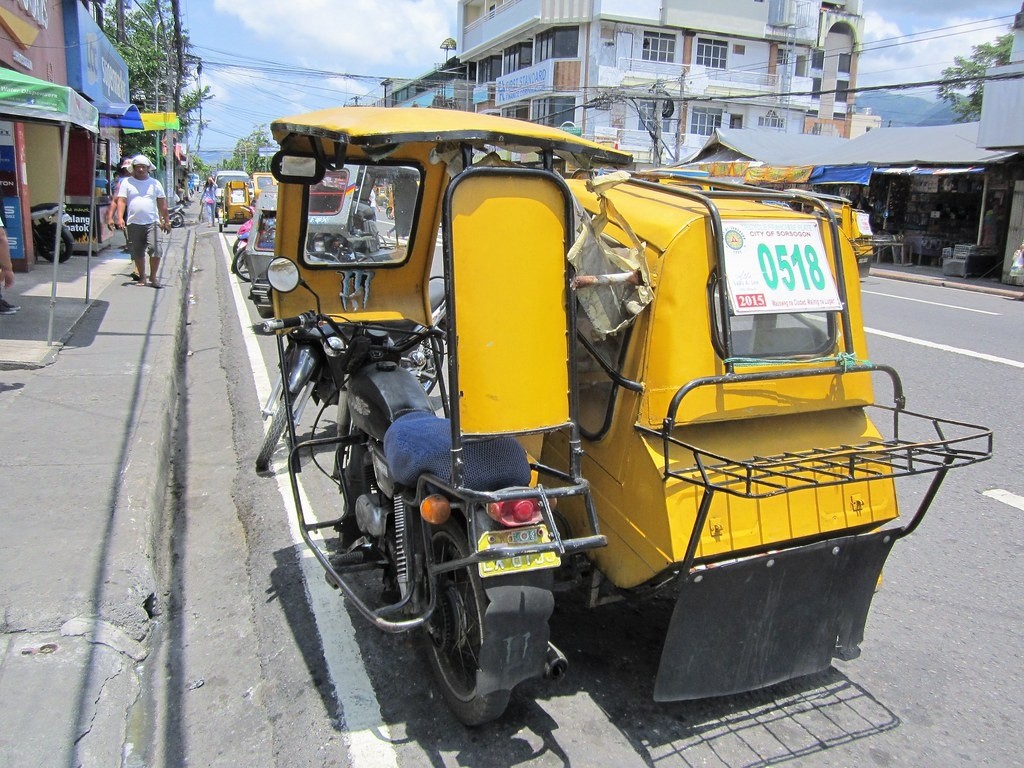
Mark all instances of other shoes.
[131,272,140,280]
[136,276,163,288]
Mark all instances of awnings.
[87,100,144,130]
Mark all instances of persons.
[0,195,21,314]
[108,155,172,290]
[176,179,193,206]
[200,177,219,227]
[369,184,380,222]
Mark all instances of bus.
[187,174,199,187]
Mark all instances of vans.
[213,171,254,217]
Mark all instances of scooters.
[217,181,253,232]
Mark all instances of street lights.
[132,18,172,182]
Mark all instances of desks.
[875,242,913,267]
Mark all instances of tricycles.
[752,189,874,278]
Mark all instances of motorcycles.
[262,257,609,728]
[233,245,252,282]
[30,203,75,263]
[233,199,256,256]
[158,204,186,228]
[255,326,444,472]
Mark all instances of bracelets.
[0,263,12,271]
[165,221,171,226]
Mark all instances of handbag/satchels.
[207,199,214,204]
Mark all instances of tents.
[0,66,100,346]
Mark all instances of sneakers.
[0,298,22,314]
